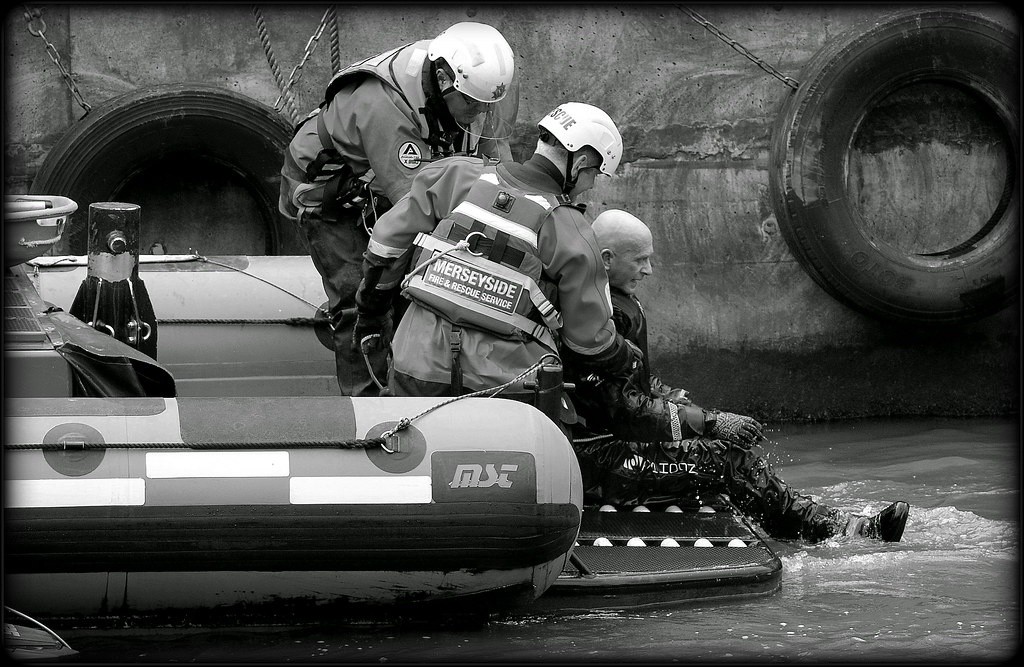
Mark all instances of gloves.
[351,312,394,353]
[715,410,764,450]
[623,339,643,374]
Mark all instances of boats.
[5,191,782,658]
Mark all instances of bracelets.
[705,412,716,432]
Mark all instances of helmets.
[428,22,515,105]
[535,102,622,178]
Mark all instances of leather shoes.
[859,501,910,542]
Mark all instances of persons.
[353,102,642,439]
[279,23,514,397]
[557,209,909,541]
